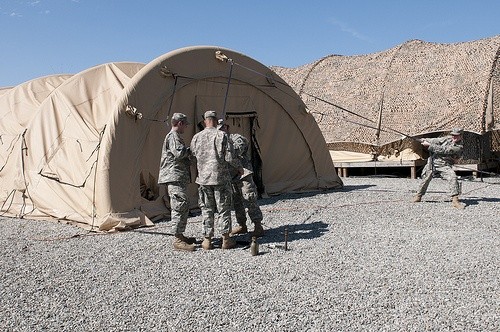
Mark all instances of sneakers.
[414,194,421,202]
[180,234,196,244]
[452,196,464,209]
[222,236,236,249]
[251,223,264,238]
[202,237,211,249]
[231,222,247,234]
[172,237,194,251]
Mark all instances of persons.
[187,110,245,250]
[215,118,265,239]
[156,112,197,251]
[413,127,465,210]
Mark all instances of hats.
[450,127,463,135]
[216,119,224,130]
[171,112,189,122]
[204,110,217,118]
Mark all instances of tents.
[266,33,499,161]
[1,46,343,236]
